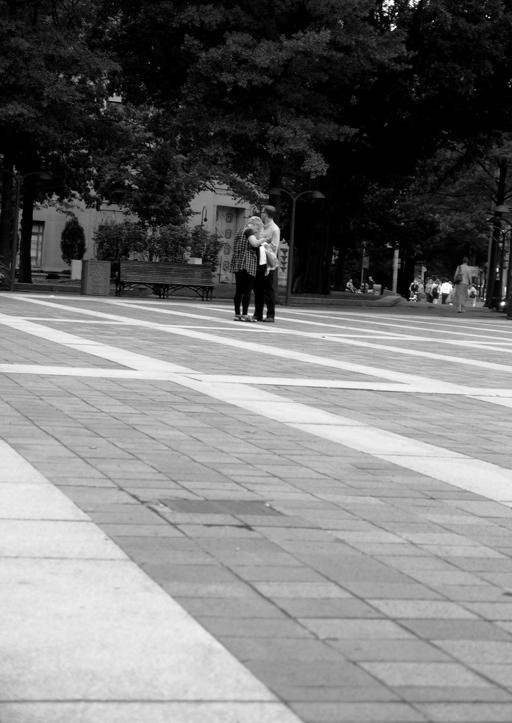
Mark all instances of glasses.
[260,211,267,213]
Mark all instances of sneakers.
[234,315,249,321]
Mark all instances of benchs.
[114,258,215,301]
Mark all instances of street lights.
[478,223,512,309]
[199,204,209,257]
[268,186,325,308]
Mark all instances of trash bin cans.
[373,284,382,295]
[70,260,110,295]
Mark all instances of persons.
[409,276,477,307]
[368,276,375,289]
[346,277,356,293]
[454,257,472,313]
[230,205,281,322]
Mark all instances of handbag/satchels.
[455,269,462,281]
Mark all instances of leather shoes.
[263,317,274,322]
